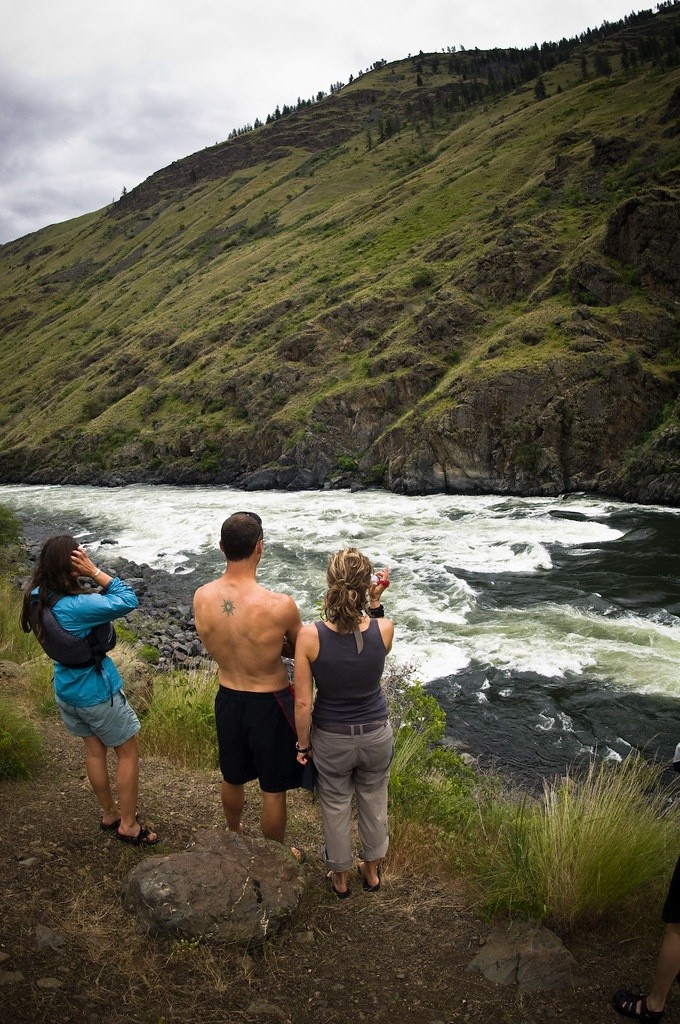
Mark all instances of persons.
[193,510,304,863]
[294,548,394,899]
[23,535,158,843]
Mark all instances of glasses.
[231,512,262,525]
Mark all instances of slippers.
[115,825,157,844]
[291,848,304,863]
[358,861,380,891]
[100,812,139,830]
[325,870,350,899]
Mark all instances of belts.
[317,720,386,736]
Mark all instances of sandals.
[613,989,665,1024]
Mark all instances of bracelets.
[91,570,101,579]
[367,606,384,618]
[296,742,312,753]
